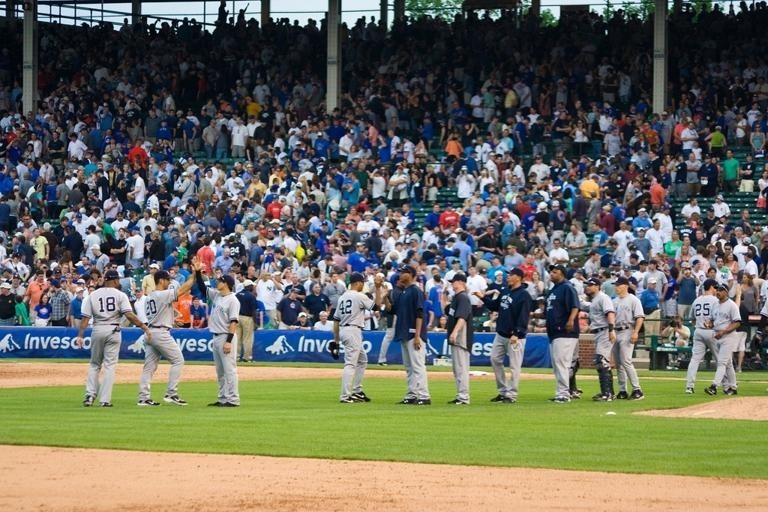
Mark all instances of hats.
[396,266,418,276]
[610,277,629,286]
[582,277,601,286]
[103,270,121,281]
[297,311,308,319]
[134,287,143,293]
[703,279,729,293]
[49,278,90,293]
[349,271,369,284]
[2,112,768,267]
[549,263,566,276]
[504,267,525,278]
[153,271,176,280]
[243,279,257,287]
[216,274,234,286]
[448,274,468,283]
[647,277,658,284]
[0,282,13,289]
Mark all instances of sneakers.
[236,356,257,362]
[448,399,469,405]
[686,387,695,394]
[340,391,371,403]
[163,395,189,406]
[98,402,114,407]
[723,388,737,396]
[83,394,94,407]
[206,402,240,407]
[547,387,583,404]
[705,385,717,397]
[593,391,644,401]
[137,399,162,406]
[489,395,516,403]
[395,398,431,406]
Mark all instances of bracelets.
[226,332,234,343]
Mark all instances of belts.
[590,326,609,335]
[213,332,230,336]
[94,323,120,326]
[148,324,171,329]
[615,325,635,332]
[698,326,722,332]
[339,323,364,331]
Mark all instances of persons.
[378,265,431,405]
[442,273,473,404]
[328,272,381,403]
[685,279,741,396]
[136,265,196,407]
[189,256,240,407]
[1,0,767,330]
[77,271,151,407]
[470,267,532,402]
[544,264,646,402]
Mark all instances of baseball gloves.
[328,342,339,360]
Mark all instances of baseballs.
[333,349,339,355]
